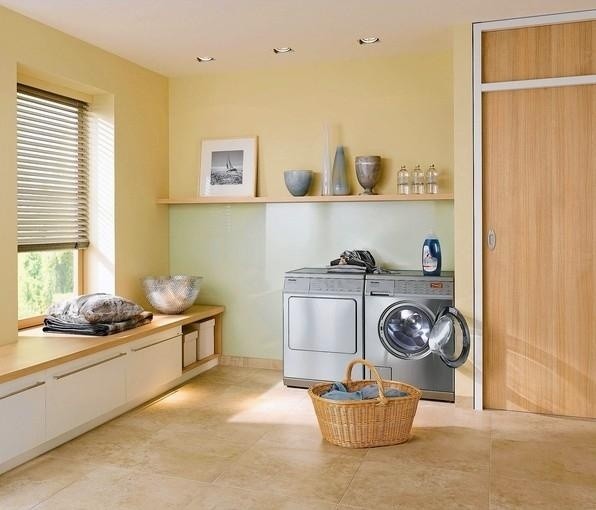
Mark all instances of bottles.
[411,165,427,193]
[397,164,410,194]
[425,163,441,192]
[331,143,351,196]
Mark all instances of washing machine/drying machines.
[282,267,364,388]
[364,270,470,403]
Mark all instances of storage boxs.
[181,329,198,368]
[189,319,216,361]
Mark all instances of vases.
[284,170,311,196]
[334,146,351,195]
[354,156,381,195]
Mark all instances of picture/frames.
[199,137,257,196]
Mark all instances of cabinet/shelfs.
[0,325,183,476]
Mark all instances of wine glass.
[353,154,382,197]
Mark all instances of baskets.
[308,359,423,449]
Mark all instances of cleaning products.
[422,231,440,276]
